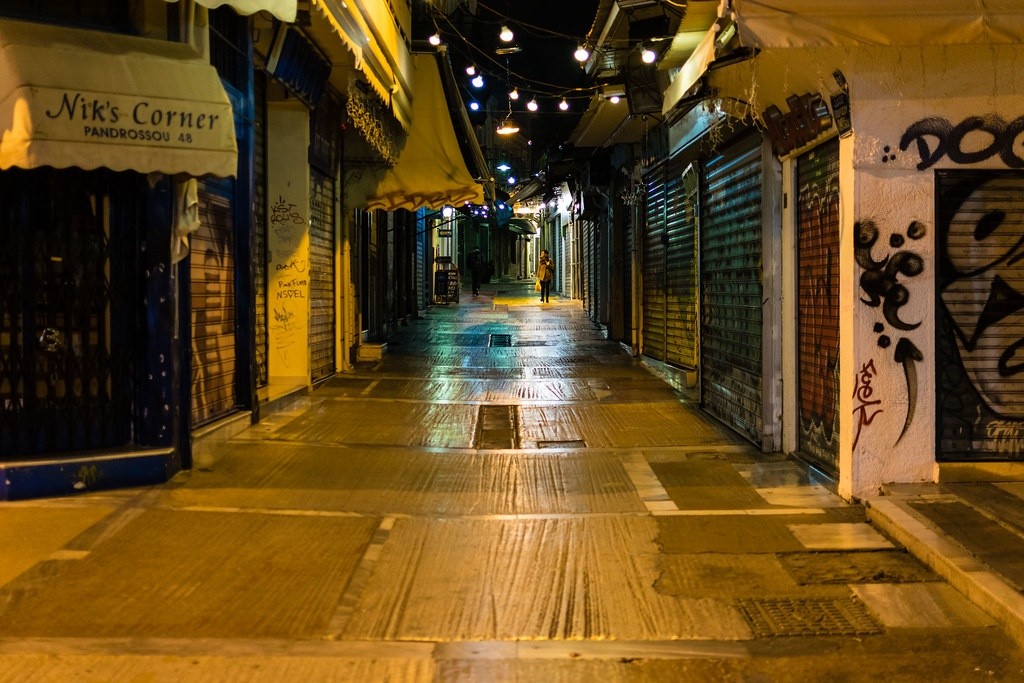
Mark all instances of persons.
[467,245,487,297]
[536,250,554,304]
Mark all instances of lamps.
[496,55,519,136]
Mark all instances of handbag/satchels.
[535,279,541,291]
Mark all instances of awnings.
[0,19,239,176]
[509,0,1023,205]
[310,0,415,133]
[341,40,496,212]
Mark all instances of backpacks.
[467,251,482,269]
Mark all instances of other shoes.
[541,299,544,302]
[473,294,476,297]
[476,289,479,296]
